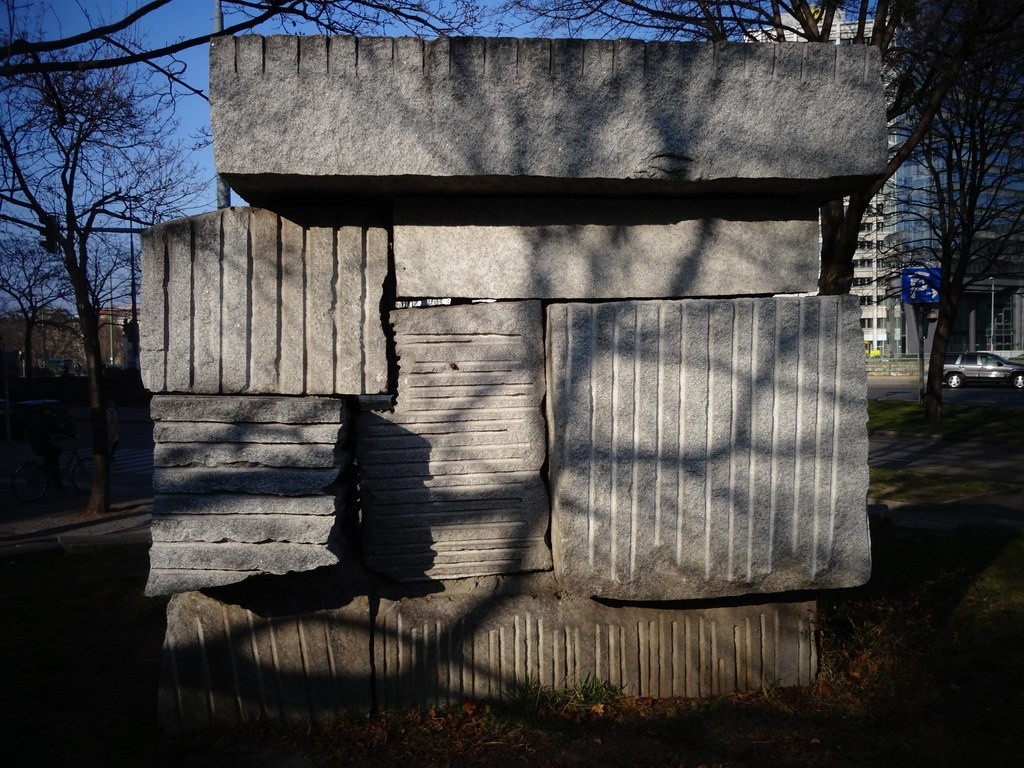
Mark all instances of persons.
[105,400,120,462]
[28,412,72,498]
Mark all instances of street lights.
[988,275,996,351]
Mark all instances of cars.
[942,352,1024,389]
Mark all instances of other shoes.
[107,454,116,462]
[55,484,71,492]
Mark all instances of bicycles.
[11,435,94,504]
